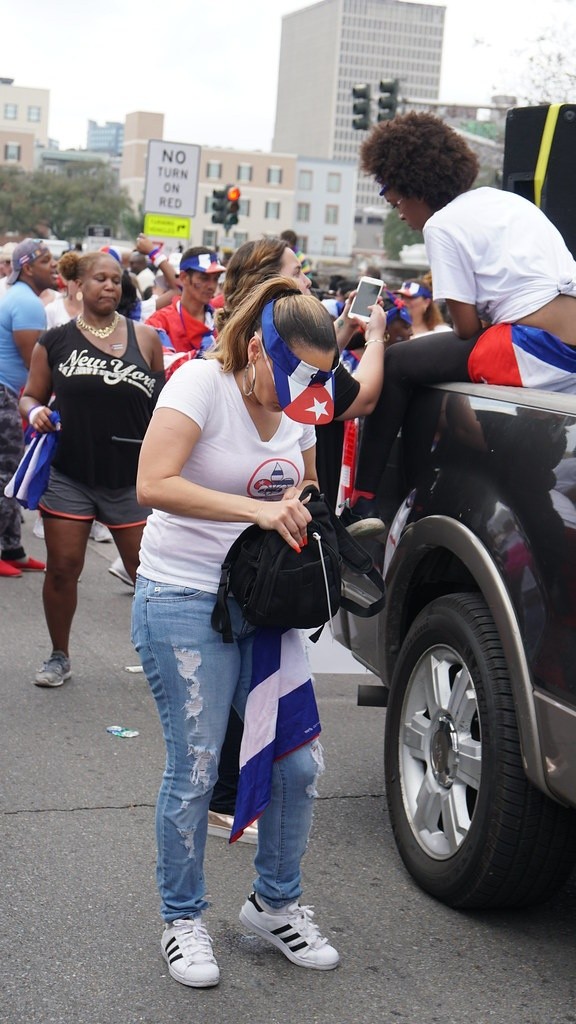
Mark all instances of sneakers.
[33,519,45,538]
[207,809,258,845]
[89,519,113,542]
[238,891,340,970]
[160,918,220,987]
[338,495,386,538]
[109,556,134,586]
[33,651,71,686]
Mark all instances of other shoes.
[3,558,45,571]
[0,559,23,577]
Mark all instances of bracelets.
[27,404,43,417]
[364,340,385,345]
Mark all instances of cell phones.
[347,276,384,323]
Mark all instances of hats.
[261,299,340,425]
[392,281,432,299]
[7,238,48,285]
[320,299,345,319]
[180,254,227,273]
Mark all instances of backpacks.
[212,483,387,643]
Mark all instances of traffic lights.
[351,83,371,130]
[222,186,240,225]
[377,78,400,121]
[211,189,225,224]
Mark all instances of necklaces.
[75,310,120,338]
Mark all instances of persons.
[31,274,113,542]
[337,112,576,539]
[131,270,343,989]
[20,246,166,691]
[107,557,134,587]
[170,238,386,847]
[0,229,453,378]
[381,386,576,706]
[0,237,59,578]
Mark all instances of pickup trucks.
[322,380,576,918]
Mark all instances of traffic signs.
[142,212,191,255]
